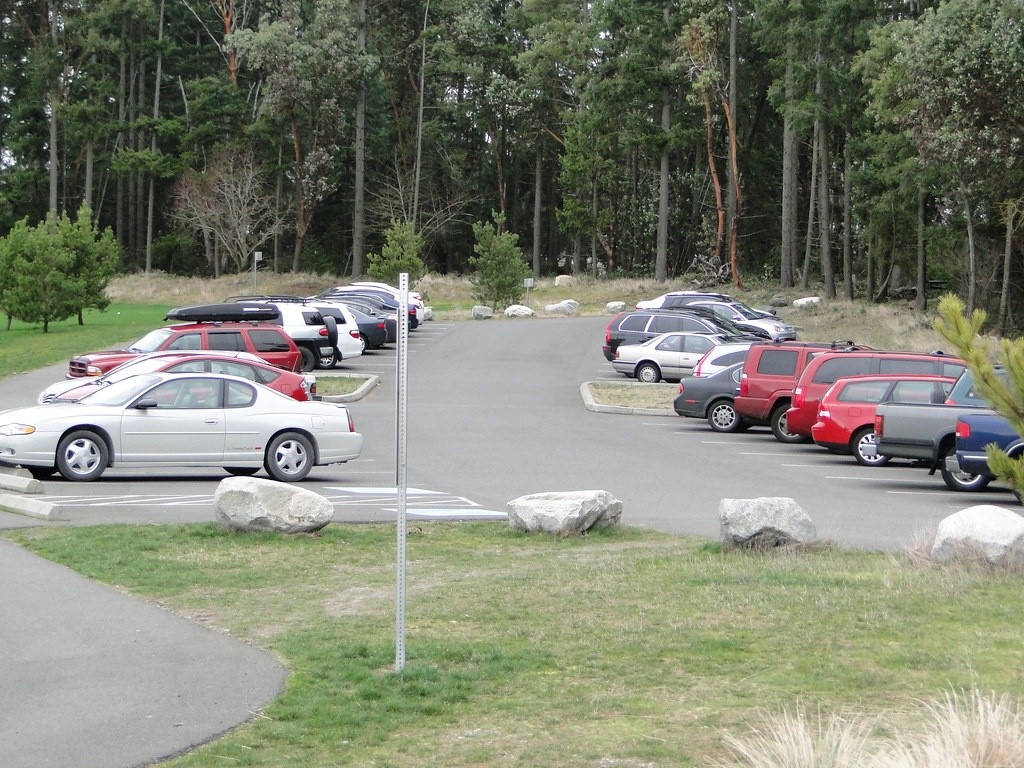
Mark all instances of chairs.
[179,337,196,350]
[183,366,192,372]
[205,390,219,408]
[179,394,196,408]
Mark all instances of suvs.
[601,310,737,362]
[299,299,362,370]
[786,345,968,456]
[735,338,874,444]
[65,322,301,379]
[223,295,338,372]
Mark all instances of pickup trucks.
[875,402,1003,493]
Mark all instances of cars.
[306,281,425,334]
[659,294,783,321]
[49,353,313,405]
[36,349,317,405]
[944,366,1009,409]
[0,371,363,483]
[635,291,774,316]
[811,374,957,467]
[686,301,796,341]
[956,414,1023,505]
[330,300,397,344]
[692,342,751,378]
[645,306,772,339]
[611,332,722,383]
[673,362,752,433]
[347,307,388,355]
